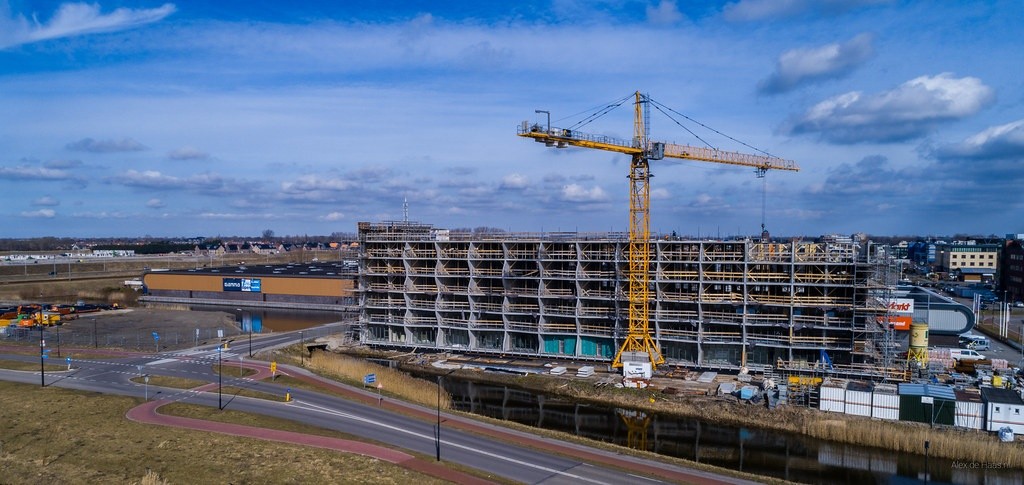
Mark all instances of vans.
[959,334,990,351]
[949,349,986,362]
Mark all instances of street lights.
[236,308,252,357]
[216,330,224,409]
[299,332,304,367]
[92,318,97,347]
[437,369,456,461]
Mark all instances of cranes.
[515,88,802,372]
